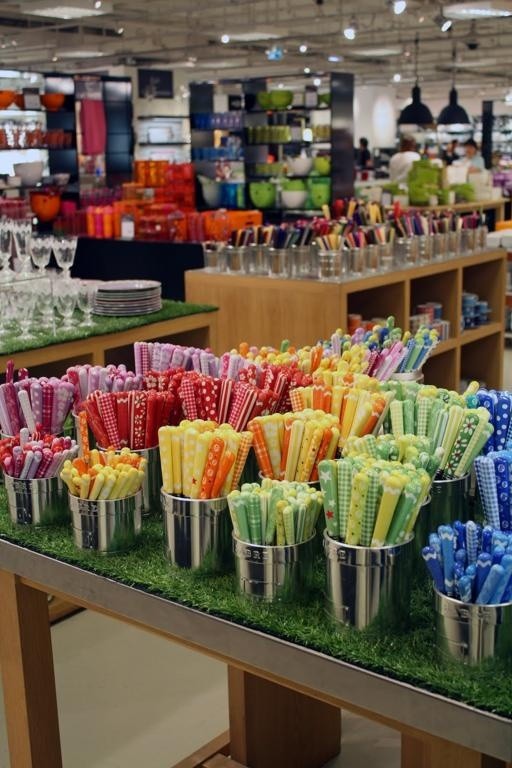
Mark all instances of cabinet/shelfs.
[135,114,190,165]
[2,72,134,210]
[243,121,289,174]
[185,246,509,398]
[188,71,356,221]
[304,82,321,107]
[0,127,75,148]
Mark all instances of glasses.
[398,1,470,124]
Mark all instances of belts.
[1,218,98,341]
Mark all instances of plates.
[5,176,23,188]
[185,246,509,398]
[91,279,163,317]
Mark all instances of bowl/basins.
[13,161,46,187]
[248,178,329,208]
[270,89,293,109]
[54,173,70,185]
[1,89,15,110]
[40,92,65,110]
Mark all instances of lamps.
[398,1,470,124]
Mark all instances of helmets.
[91,279,163,317]
[5,176,23,188]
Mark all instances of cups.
[432,579,510,664]
[229,534,316,601]
[257,469,320,490]
[65,486,145,556]
[160,484,233,573]
[347,299,451,345]
[3,469,65,528]
[417,476,468,539]
[323,526,414,633]
[201,223,488,281]
[96,440,159,511]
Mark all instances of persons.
[388,132,421,184]
[354,136,370,168]
[443,138,459,164]
[462,138,485,168]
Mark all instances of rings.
[201,196,488,279]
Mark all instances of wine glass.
[1,218,98,341]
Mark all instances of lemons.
[284,147,313,176]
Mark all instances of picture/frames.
[383,198,506,232]
[137,70,174,100]
[0,540,512,767]
[0,298,221,373]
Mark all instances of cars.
[201,223,488,281]
[347,299,451,345]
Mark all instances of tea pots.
[284,147,313,176]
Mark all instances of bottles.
[0,127,75,148]
[243,121,289,174]
[304,82,321,107]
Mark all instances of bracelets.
[40,92,65,110]
[54,173,70,185]
[270,89,293,109]
[1,89,15,110]
[13,161,46,187]
[248,178,329,208]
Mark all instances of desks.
[0,540,512,767]
[0,298,221,373]
[383,198,506,232]
